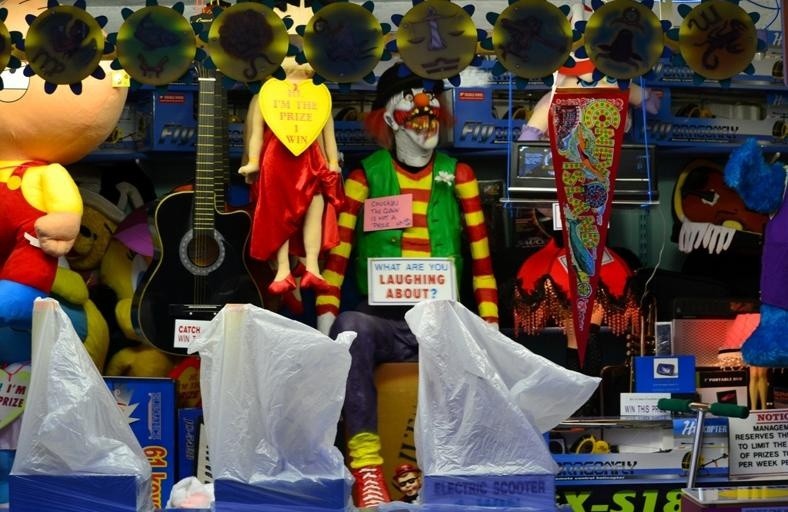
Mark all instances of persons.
[391,462,423,504]
[313,62,502,509]
[515,206,650,374]
[234,2,344,312]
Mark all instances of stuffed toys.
[208,1,787,510]
[0,0,201,411]
[518,2,661,143]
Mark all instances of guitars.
[131,14,262,357]
